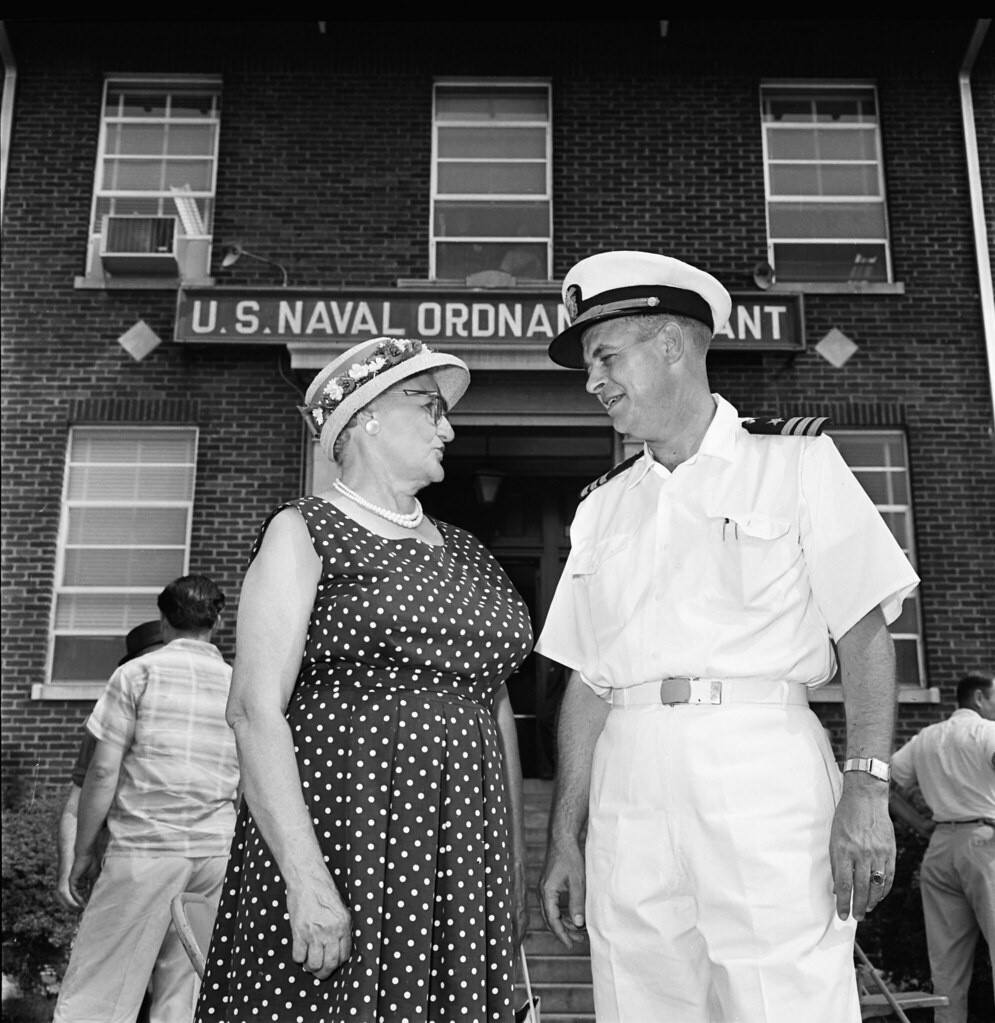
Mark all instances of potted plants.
[0,796,108,1023]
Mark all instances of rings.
[871,870,886,888]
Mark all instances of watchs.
[843,758,892,784]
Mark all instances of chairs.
[846,943,948,1023]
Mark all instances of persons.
[887,669,995,1023]
[49,572,240,1023]
[191,337,536,1023]
[538,249,921,1023]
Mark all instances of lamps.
[748,261,774,290]
[221,241,289,288]
[471,435,506,505]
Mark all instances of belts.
[936,818,995,827]
[609,678,811,708]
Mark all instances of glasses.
[386,388,445,424]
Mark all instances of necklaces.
[331,477,424,530]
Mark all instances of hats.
[548,250,733,370]
[117,619,171,664]
[306,336,470,459]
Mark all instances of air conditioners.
[101,212,182,273]
